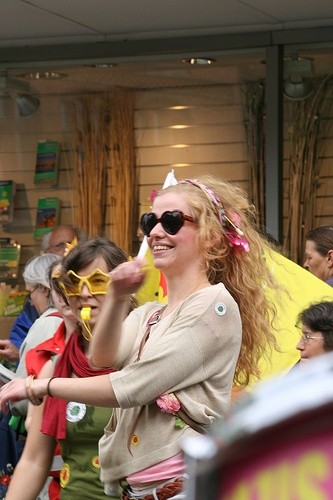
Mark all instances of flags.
[130,171,333,389]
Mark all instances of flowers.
[156,393,181,415]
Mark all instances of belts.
[121,477,184,500]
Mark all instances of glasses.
[58,269,111,296]
[140,210,198,238]
[302,334,325,344]
[26,285,38,302]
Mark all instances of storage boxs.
[0,179,17,224]
[32,197,62,242]
[33,139,62,184]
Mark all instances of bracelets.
[46,376,55,397]
[25,374,43,406]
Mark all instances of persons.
[0,225,333,500]
[0,175,287,500]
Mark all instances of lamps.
[282,57,316,101]
[15,95,42,120]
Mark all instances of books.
[0,141,61,317]
